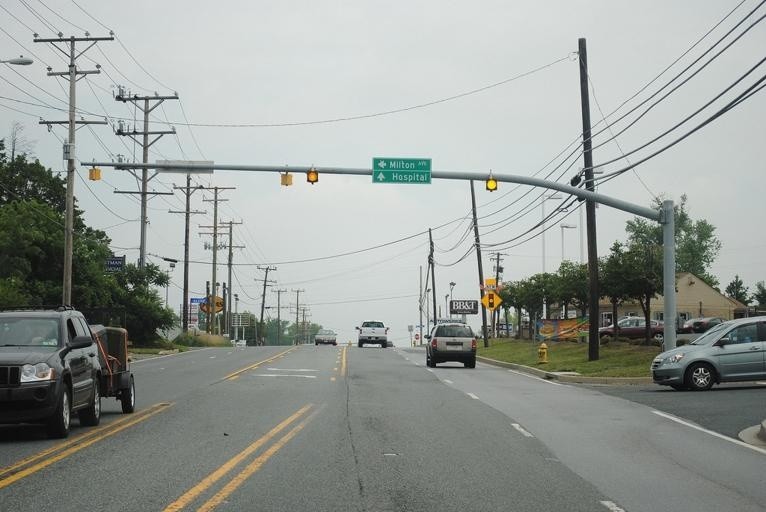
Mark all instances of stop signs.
[414,333,419,340]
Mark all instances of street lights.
[233,292,240,341]
[426,288,432,335]
[0,56,35,71]
[449,281,456,323]
[215,281,222,336]
[560,222,578,264]
[445,294,449,317]
[165,261,176,308]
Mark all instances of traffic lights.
[489,293,494,308]
[486,178,498,192]
[306,170,318,183]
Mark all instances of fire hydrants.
[537,343,549,364]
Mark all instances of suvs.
[355,321,390,348]
[424,322,478,369]
[0,303,106,437]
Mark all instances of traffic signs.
[371,157,432,184]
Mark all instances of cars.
[187,324,200,335]
[313,329,338,346]
[597,314,766,393]
